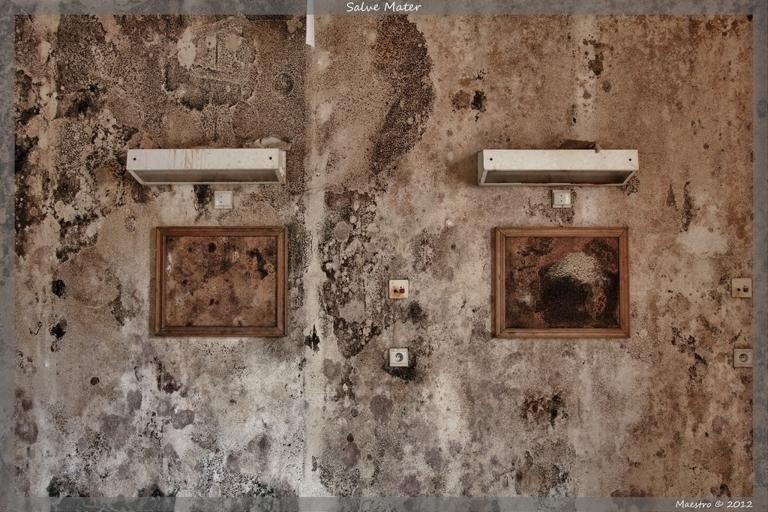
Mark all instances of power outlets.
[732,277,753,297]
[214,191,232,209]
[389,348,408,367]
[552,189,572,209]
[734,348,754,368]
[389,280,409,299]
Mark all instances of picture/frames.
[155,226,287,337]
[494,226,629,338]
